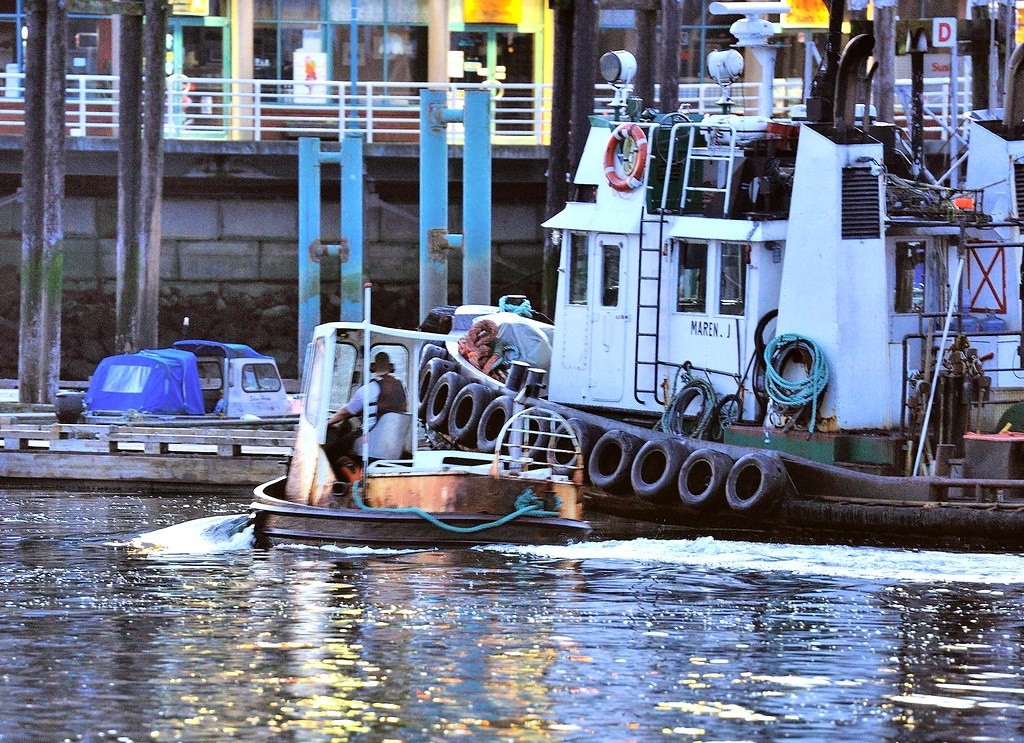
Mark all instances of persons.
[326,351,409,465]
[213,389,224,414]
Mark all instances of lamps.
[599,50,637,83]
[706,49,744,81]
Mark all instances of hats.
[218,390,224,395]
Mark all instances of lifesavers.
[415,343,788,517]
[603,123,647,191]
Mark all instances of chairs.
[352,410,413,460]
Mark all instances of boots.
[335,456,363,483]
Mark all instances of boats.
[250,0,1024,559]
